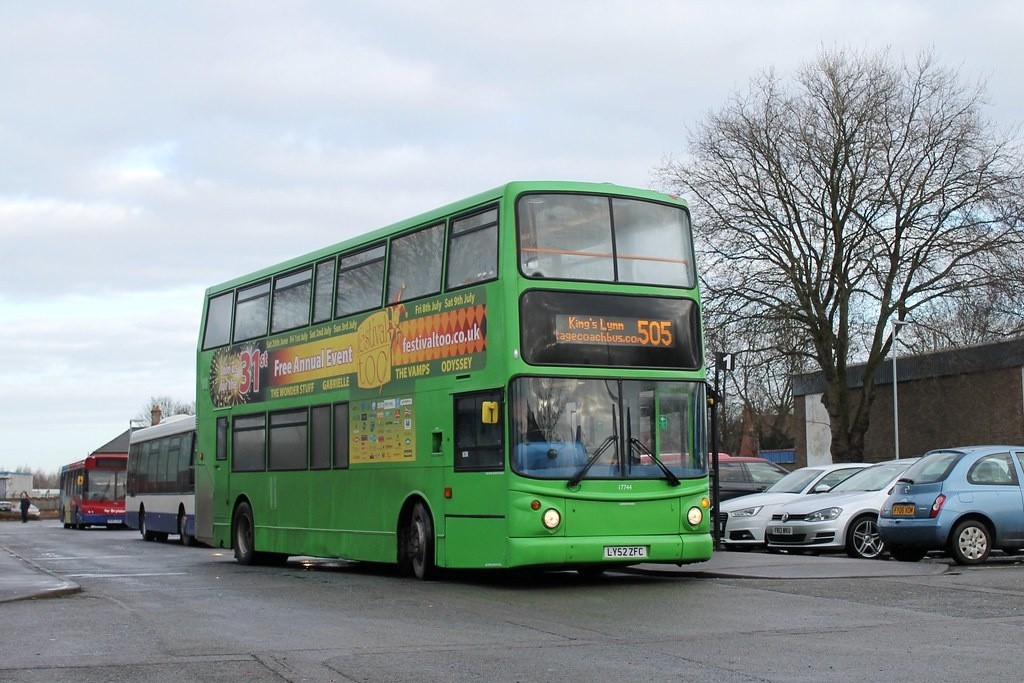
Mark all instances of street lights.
[891,318,909,460]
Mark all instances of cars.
[641,451,791,508]
[766,456,922,560]
[11,502,41,520]
[708,463,875,553]
[879,445,1024,566]
[0,502,11,512]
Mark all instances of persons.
[513,396,528,443]
[21,494,31,523]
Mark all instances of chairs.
[975,463,993,482]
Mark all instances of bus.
[192,180,712,583]
[124,414,196,547]
[58,453,128,531]
